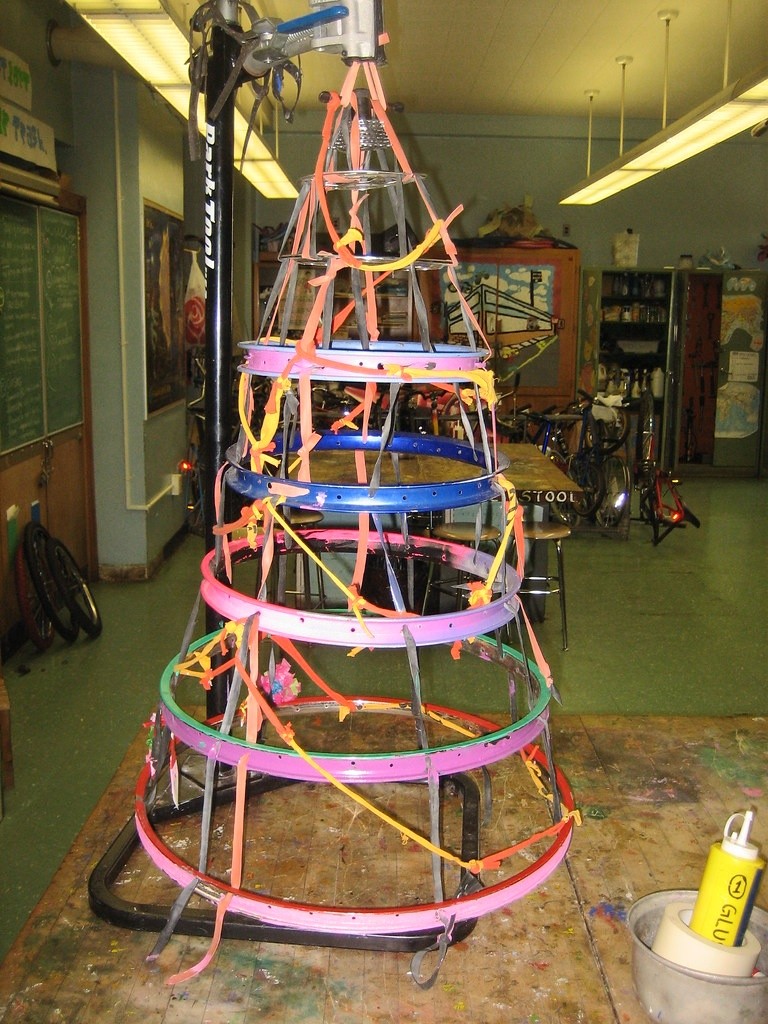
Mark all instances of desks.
[275,443,583,622]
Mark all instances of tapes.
[650,901,761,978]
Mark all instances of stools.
[250,509,328,650]
[505,523,572,651]
[420,521,514,645]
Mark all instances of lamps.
[557,0,768,205]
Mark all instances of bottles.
[621,303,659,321]
[598,364,606,391]
[453,420,464,440]
[689,812,766,947]
[650,368,664,398]
[613,272,652,297]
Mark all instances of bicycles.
[175,358,662,537]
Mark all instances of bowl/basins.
[626,889,768,1024]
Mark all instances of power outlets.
[173,474,183,496]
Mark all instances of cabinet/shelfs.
[412,243,582,443]
[595,268,768,480]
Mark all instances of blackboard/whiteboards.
[0,194,85,454]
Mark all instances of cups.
[680,255,692,268]
